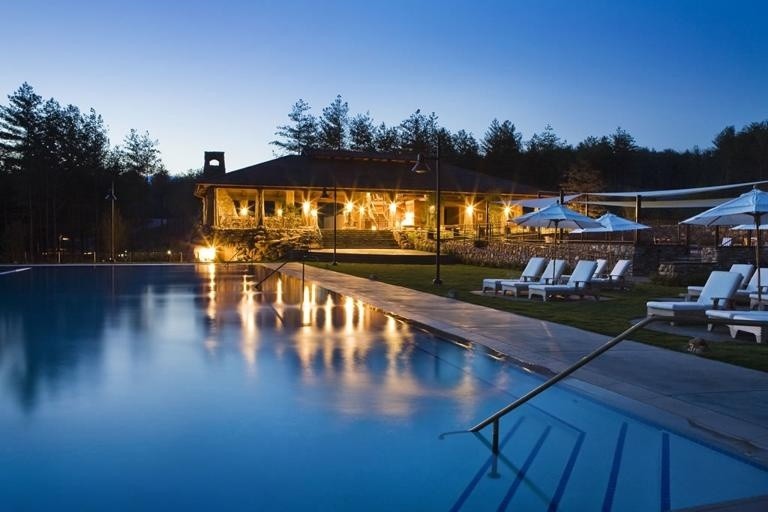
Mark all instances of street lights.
[320,175,337,265]
[105,180,120,263]
[411,135,441,287]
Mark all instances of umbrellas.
[569,209,652,277]
[729,225,768,267]
[678,183,768,311]
[506,199,606,285]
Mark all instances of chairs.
[646,271,744,327]
[483,257,547,295]
[705,309,768,344]
[687,264,754,306]
[500,259,568,297]
[733,268,768,310]
[559,258,631,293]
[528,259,598,302]
